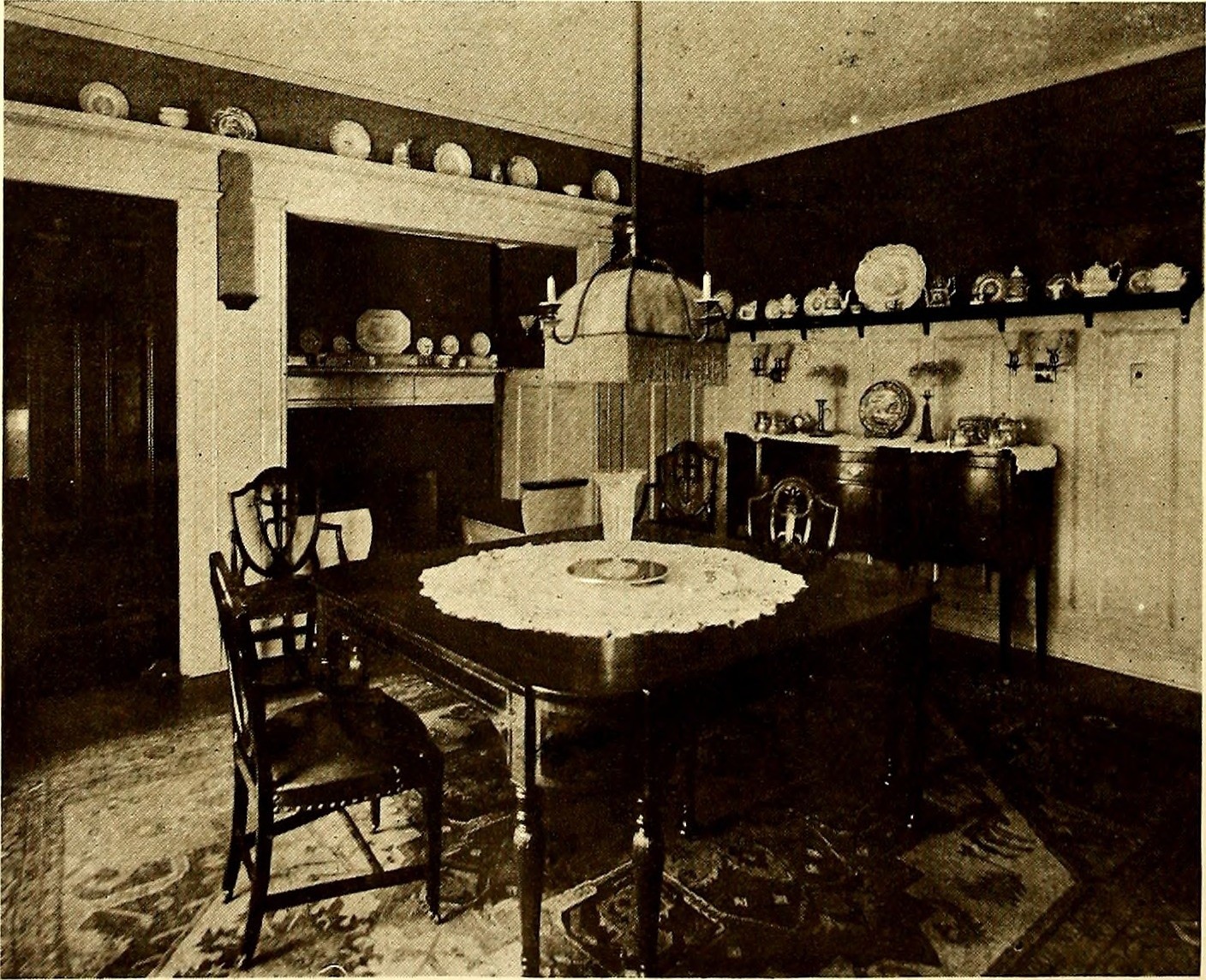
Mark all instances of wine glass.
[594,470,647,576]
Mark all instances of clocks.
[857,379,916,440]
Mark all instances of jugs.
[1129,262,1188,293]
[754,410,825,437]
[956,412,1028,447]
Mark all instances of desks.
[305,519,937,978]
[724,431,1059,684]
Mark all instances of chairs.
[228,466,369,694]
[634,440,720,535]
[209,550,446,973]
[748,476,840,560]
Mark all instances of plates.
[432,142,472,177]
[858,378,912,435]
[507,155,538,189]
[210,105,257,141]
[567,559,669,585]
[853,243,926,313]
[328,120,371,160]
[77,81,129,119]
[592,169,619,202]
[300,310,491,368]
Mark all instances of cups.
[158,107,189,128]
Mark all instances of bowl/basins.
[562,184,581,197]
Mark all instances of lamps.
[531,1,731,387]
[1000,328,1073,380]
[747,342,793,379]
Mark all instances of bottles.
[391,138,412,169]
[490,156,504,183]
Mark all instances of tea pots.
[922,272,958,308]
[997,265,1031,302]
[1065,260,1123,297]
[808,281,851,316]
